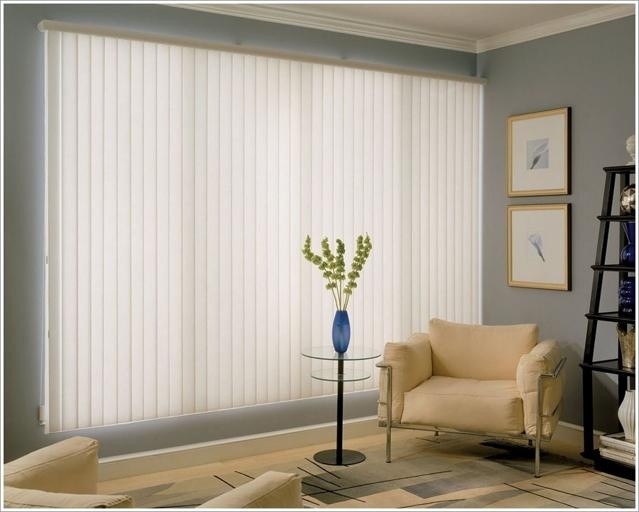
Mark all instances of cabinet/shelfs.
[577,166,636,482]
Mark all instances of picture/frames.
[505,106,573,292]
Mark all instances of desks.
[300,345,382,466]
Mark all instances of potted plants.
[300,233,374,354]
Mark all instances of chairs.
[375,317,567,478]
[1,435,303,508]
